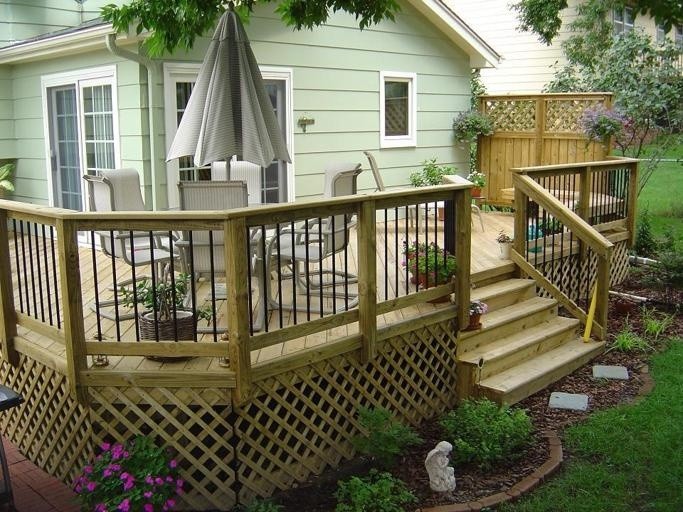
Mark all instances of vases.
[497,240,511,260]
[470,185,480,198]
[409,270,449,303]
[464,315,480,330]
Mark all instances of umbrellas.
[164,0,292,182]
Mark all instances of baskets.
[138,306,197,362]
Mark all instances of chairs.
[363,150,485,239]
[175,181,269,334]
[211,161,261,205]
[83,167,183,322]
[265,162,361,314]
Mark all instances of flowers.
[401,243,459,282]
[465,169,485,189]
[73,436,184,511]
[495,229,511,242]
[465,300,487,314]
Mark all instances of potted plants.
[115,272,215,365]
[530,218,560,253]
